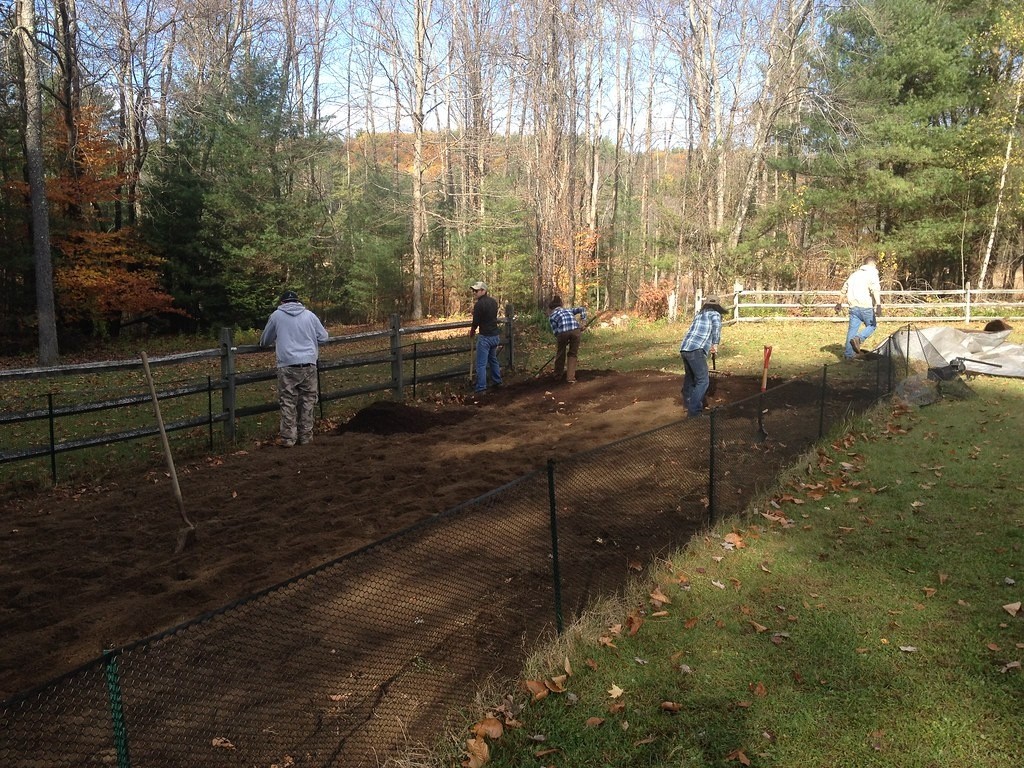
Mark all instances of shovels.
[757,345,773,441]
[140,350,197,553]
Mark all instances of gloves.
[834,302,842,315]
[470,329,475,338]
[874,305,881,317]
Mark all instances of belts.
[289,363,315,368]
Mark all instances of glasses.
[472,290,478,293]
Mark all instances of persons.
[549,296,586,383]
[680,296,726,418]
[260,290,328,446]
[469,282,504,396]
[835,256,881,358]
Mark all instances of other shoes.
[487,381,503,388]
[464,385,487,394]
[850,337,861,354]
[301,436,314,445]
[275,436,294,447]
[567,377,577,383]
[552,373,561,384]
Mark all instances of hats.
[703,294,721,306]
[470,282,488,291]
[549,296,564,306]
[281,291,299,301]
